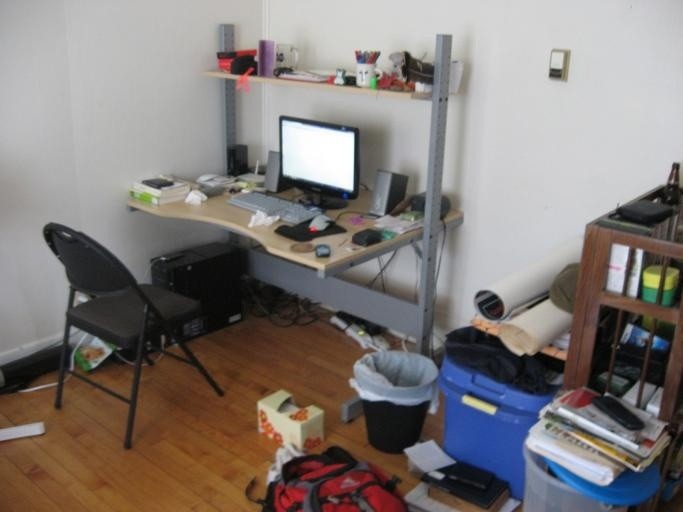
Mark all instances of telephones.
[195,172,236,188]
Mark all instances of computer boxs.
[150,240,243,350]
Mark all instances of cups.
[274,43,299,73]
[354,61,384,87]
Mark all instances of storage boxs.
[522,439,658,511]
[436,350,563,504]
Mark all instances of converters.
[346,323,372,348]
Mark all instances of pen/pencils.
[355,50,381,63]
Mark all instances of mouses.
[309,214,334,231]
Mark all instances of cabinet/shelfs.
[563,187,683,512]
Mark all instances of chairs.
[40,220,225,449]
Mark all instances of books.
[129,175,189,206]
[525,390,671,487]
[403,463,521,511]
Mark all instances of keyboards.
[226,191,323,224]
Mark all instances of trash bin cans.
[355,350,440,454]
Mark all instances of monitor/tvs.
[278,115,360,208]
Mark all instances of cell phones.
[593,396,647,430]
[316,244,330,257]
[159,251,184,261]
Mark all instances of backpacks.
[258,444,409,512]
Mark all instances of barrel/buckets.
[522,434,630,512]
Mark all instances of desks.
[126,164,465,426]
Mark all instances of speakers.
[263,151,293,193]
[370,169,408,216]
